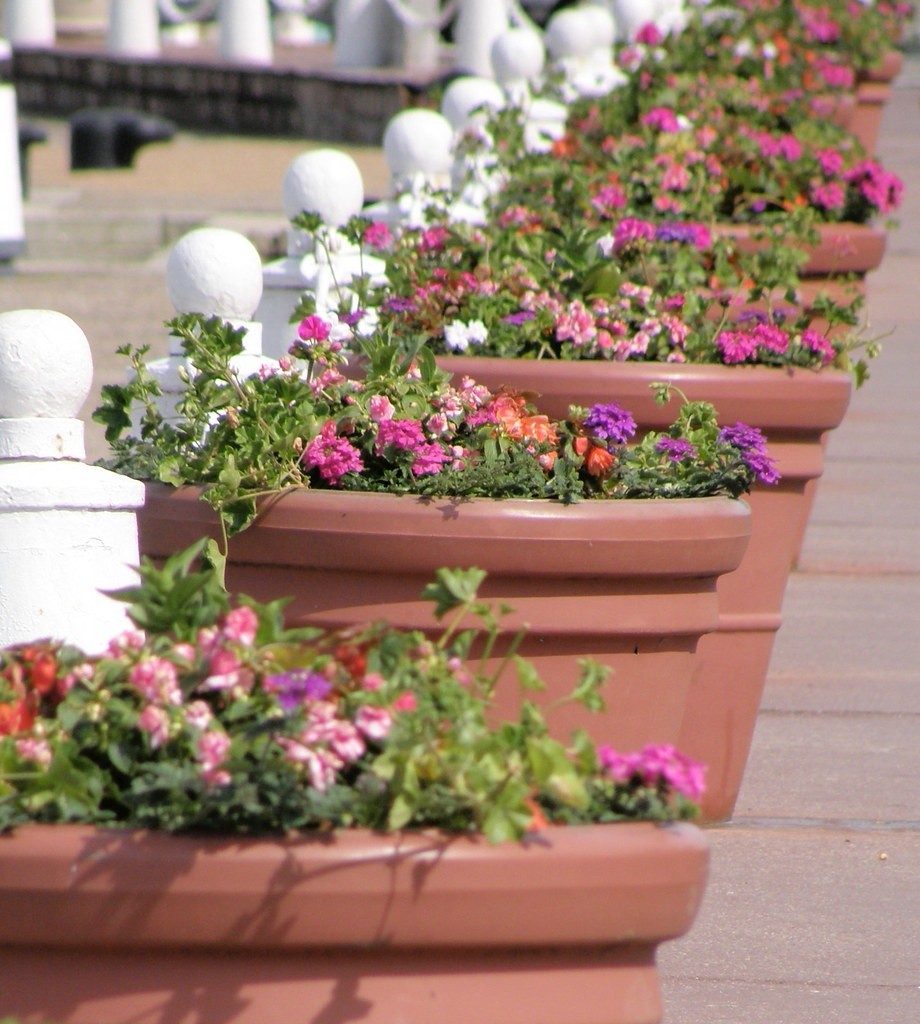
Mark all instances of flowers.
[0,2,917,833]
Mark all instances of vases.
[711,224,891,451]
[840,86,889,161]
[853,50,907,89]
[425,356,853,571]
[0,822,713,1024]
[136,485,753,749]
[663,462,823,827]
